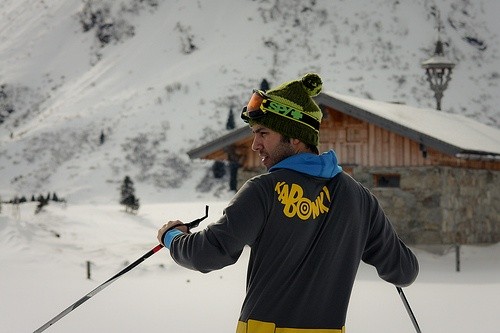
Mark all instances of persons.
[158,73,419,333]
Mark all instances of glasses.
[246,89,271,117]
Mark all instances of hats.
[241,73,323,146]
